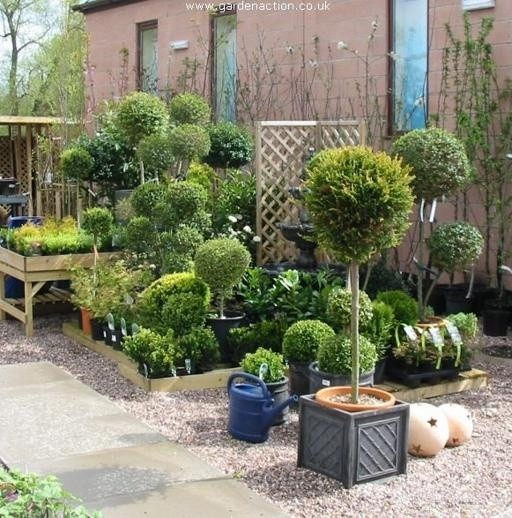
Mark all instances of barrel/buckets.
[266,376,291,426]
[4,216,54,299]
[287,359,312,403]
[307,359,377,393]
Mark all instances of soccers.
[441,404,472,447]
[407,403,448,458]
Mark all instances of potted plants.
[3,87,493,491]
